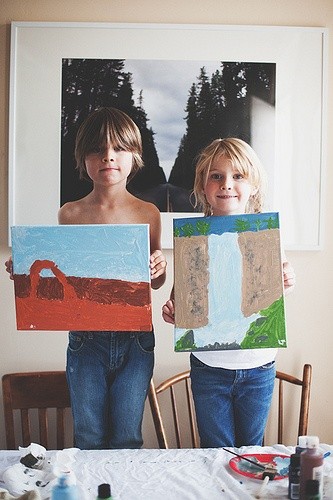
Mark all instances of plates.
[229,453,291,480]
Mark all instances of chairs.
[2,370,76,451]
[148,364,311,450]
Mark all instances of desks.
[0,444,333,500]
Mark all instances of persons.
[162,137,295,448]
[5,108,167,450]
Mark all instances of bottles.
[94,484,113,500]
[52,477,74,500]
[289,435,323,500]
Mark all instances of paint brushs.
[223,448,265,469]
[323,452,330,459]
[255,472,271,499]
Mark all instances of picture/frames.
[8,20,329,253]
